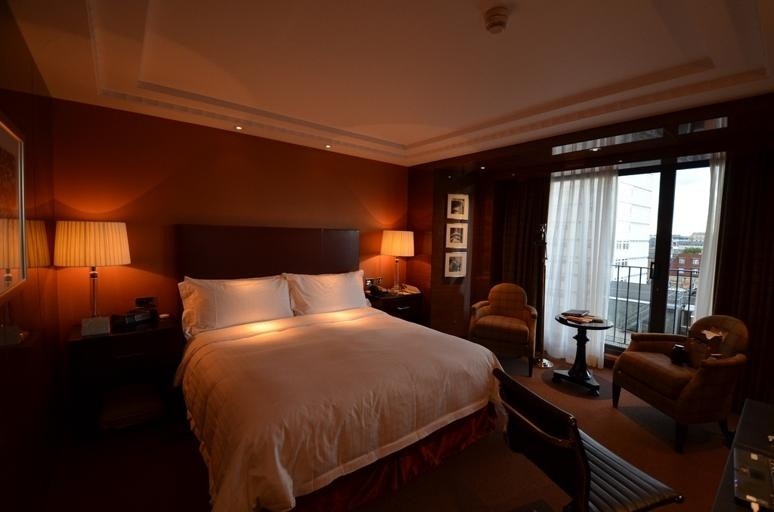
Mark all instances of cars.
[678,288,686,292]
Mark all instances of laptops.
[731,445,774,512]
[561,310,590,316]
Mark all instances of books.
[560,308,603,325]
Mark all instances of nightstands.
[63,318,177,400]
[367,291,424,323]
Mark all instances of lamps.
[53,220,131,332]
[0,219,51,346]
[380,230,414,290]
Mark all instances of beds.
[166,223,495,512]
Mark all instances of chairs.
[467,283,538,378]
[612,315,750,452]
[492,368,685,512]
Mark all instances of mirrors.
[0,110,31,301]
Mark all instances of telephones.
[369,285,388,296]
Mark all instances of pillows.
[281,270,372,316]
[177,274,294,340]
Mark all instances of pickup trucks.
[687,287,697,295]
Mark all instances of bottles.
[670,344,685,365]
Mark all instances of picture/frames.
[443,192,470,280]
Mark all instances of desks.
[711,398,774,512]
[552,313,614,397]
